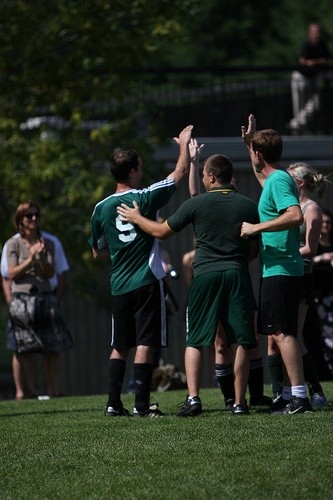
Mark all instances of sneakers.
[273,395,293,409]
[250,395,271,405]
[224,398,248,409]
[231,402,250,415]
[281,396,314,414]
[105,405,133,417]
[134,402,164,418]
[177,394,201,416]
[310,392,327,409]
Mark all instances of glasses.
[24,212,41,219]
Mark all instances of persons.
[240,114,318,416]
[91,125,272,418]
[117,156,261,416]
[0,228,71,398]
[5,201,67,399]
[287,22,333,135]
[269,161,333,409]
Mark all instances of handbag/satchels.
[150,365,187,392]
